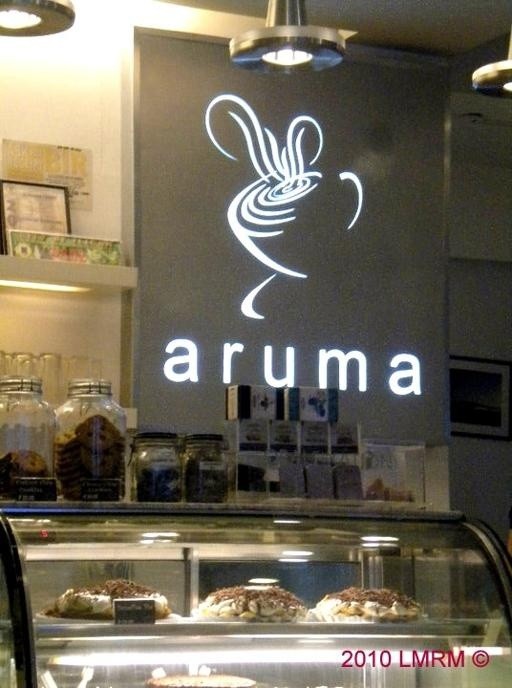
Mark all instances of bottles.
[0,376,230,502]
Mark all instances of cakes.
[307,587,431,625]
[49,582,173,623]
[190,582,315,625]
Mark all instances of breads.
[146,672,256,687]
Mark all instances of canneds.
[130,433,183,501]
[183,433,227,501]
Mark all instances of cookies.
[52,414,125,503]
[0,450,56,500]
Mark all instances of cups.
[0,352,63,401]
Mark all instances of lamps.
[1,0,510,98]
